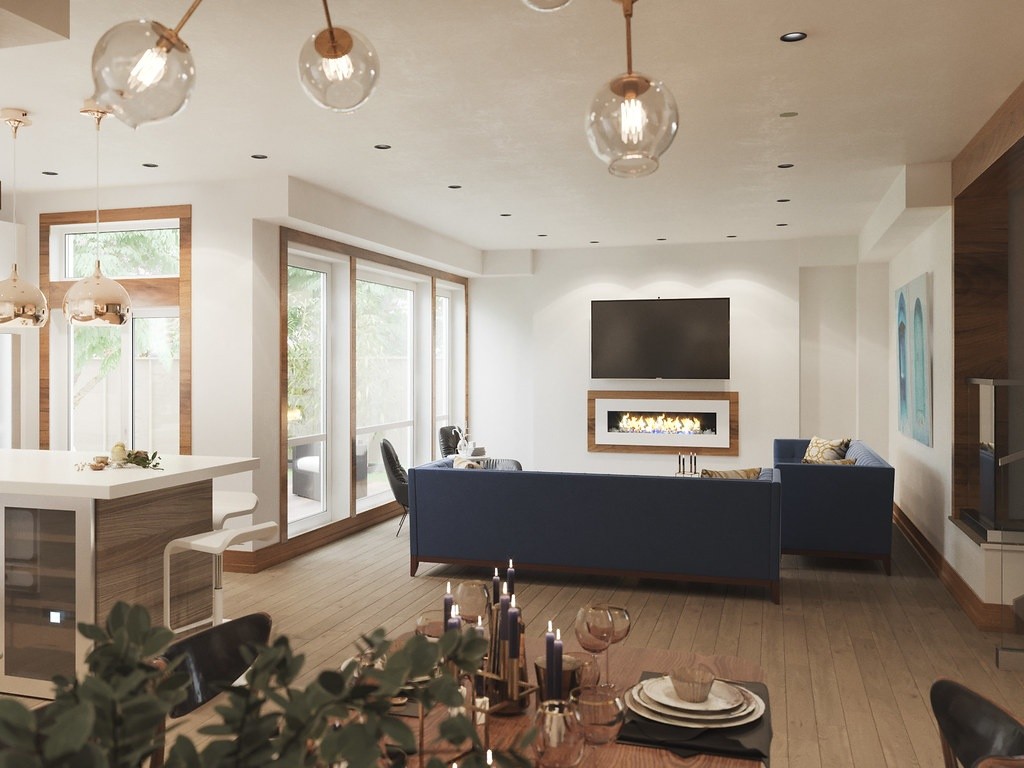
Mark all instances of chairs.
[380,438,408,537]
[440,426,461,457]
[930,679,1024,768]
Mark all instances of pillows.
[805,436,852,457]
[701,468,763,480]
[451,458,483,471]
[802,458,857,465]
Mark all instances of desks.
[276,663,771,768]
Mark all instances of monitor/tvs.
[591,298,731,379]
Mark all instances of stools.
[212,493,259,530]
[164,522,279,686]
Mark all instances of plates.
[625,674,766,728]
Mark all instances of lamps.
[91,0,202,127]
[0,109,49,329]
[299,0,379,112]
[61,100,131,326]
[584,0,679,178]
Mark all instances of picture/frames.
[587,390,739,456]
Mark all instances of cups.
[670,668,715,703]
[456,579,488,624]
[416,608,461,644]
[590,600,631,644]
[529,699,584,768]
[569,685,624,743]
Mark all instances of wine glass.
[576,607,615,695]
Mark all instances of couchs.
[408,457,781,604]
[774,438,895,575]
